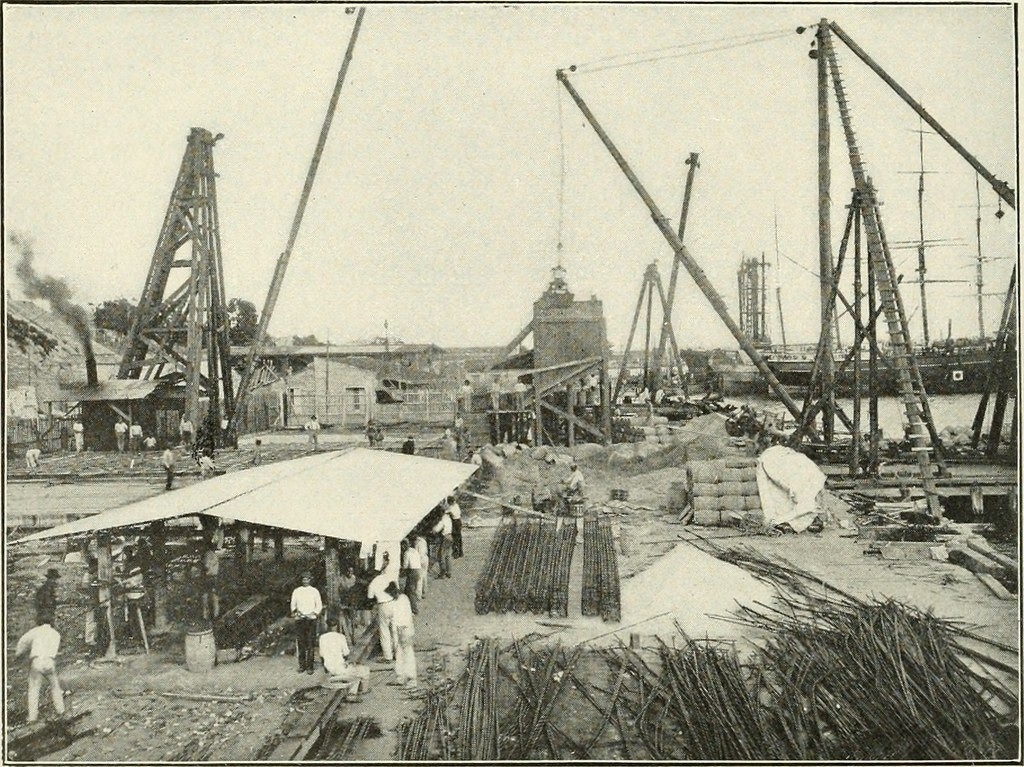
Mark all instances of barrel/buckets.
[184,628,215,672]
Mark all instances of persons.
[365,421,384,448]
[199,449,214,481]
[305,415,321,452]
[162,442,176,490]
[319,617,372,703]
[290,571,323,675]
[82,520,245,643]
[565,463,585,493]
[35,569,60,625]
[402,436,414,454]
[253,440,262,465]
[73,419,84,452]
[448,377,537,477]
[144,434,156,450]
[179,415,193,444]
[17,613,66,721]
[114,417,129,452]
[25,448,43,467]
[130,420,143,455]
[339,496,463,681]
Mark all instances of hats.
[385,582,401,594]
[45,570,61,578]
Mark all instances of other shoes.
[386,681,400,686]
[307,668,314,675]
[402,685,417,690]
[296,667,304,673]
